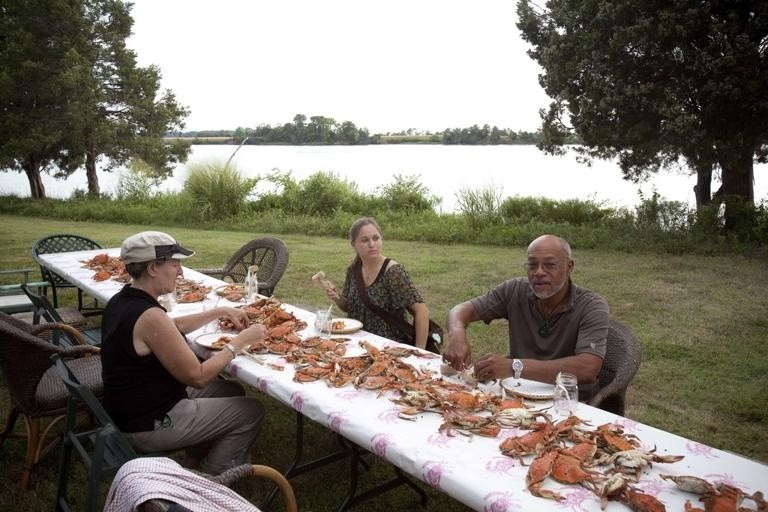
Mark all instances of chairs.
[502,319,642,418]
[1,233,289,511]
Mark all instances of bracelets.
[226,342,237,360]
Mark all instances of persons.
[100,230,267,477]
[326,216,431,350]
[443,233,610,402]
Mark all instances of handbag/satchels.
[412,315,443,354]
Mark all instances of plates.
[194,331,239,351]
[327,317,364,334]
[499,376,554,400]
[216,285,245,295]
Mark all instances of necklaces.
[538,299,564,338]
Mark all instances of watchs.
[512,357,524,379]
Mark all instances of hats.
[121,231,196,265]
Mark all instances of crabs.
[174,276,246,304]
[210,296,768,512]
[78,252,134,284]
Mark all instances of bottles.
[245,277,258,301]
[316,310,332,338]
[554,372,579,415]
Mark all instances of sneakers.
[184,446,201,466]
